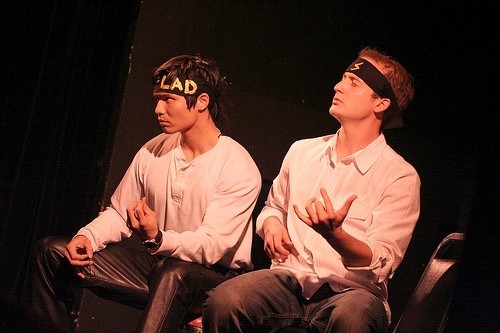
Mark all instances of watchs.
[143,229,162,250]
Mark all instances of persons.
[13,55,262,333]
[201,49,421,333]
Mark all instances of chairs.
[393,232,465,333]
